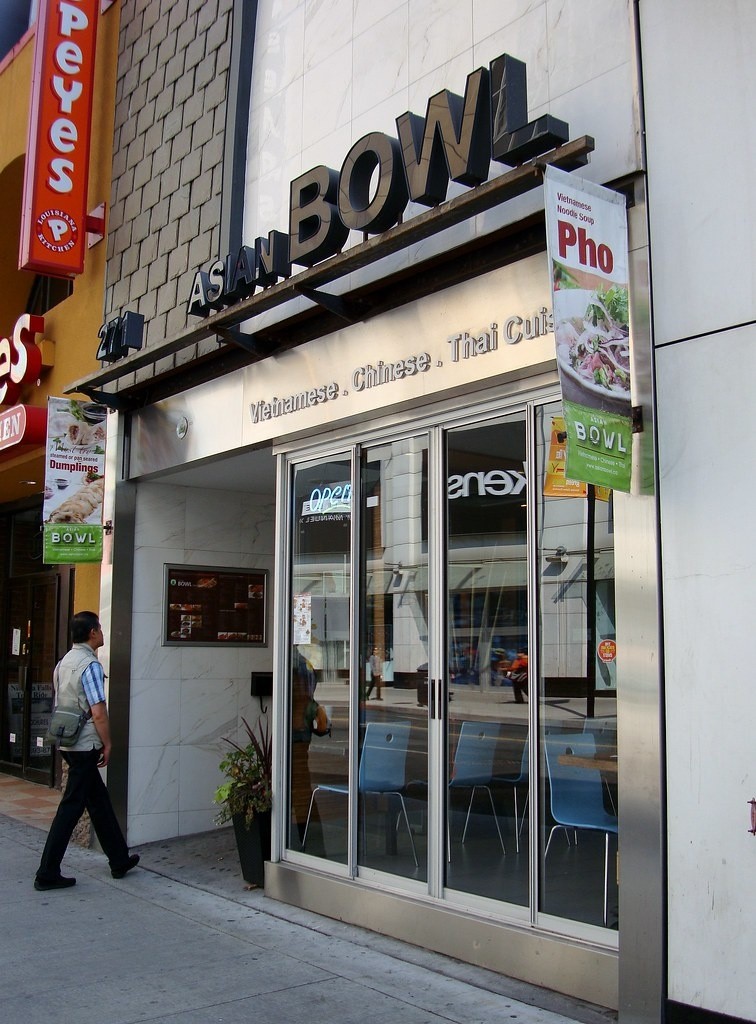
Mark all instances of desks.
[296,721,617,780]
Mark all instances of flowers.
[215,715,271,831]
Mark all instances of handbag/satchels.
[44,705,88,747]
[507,670,521,681]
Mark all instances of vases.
[233,812,270,888]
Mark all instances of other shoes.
[376,697,384,701]
[367,695,369,700]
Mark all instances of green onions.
[591,340,629,387]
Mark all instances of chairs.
[488,733,535,853]
[300,721,424,868]
[544,735,617,926]
[392,722,507,853]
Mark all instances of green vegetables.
[583,282,628,332]
[52,399,105,482]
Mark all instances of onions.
[576,299,630,380]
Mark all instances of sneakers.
[34,875,76,891]
[112,854,140,878]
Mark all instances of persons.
[291,625,332,860]
[366,648,383,701]
[503,649,528,704]
[34,610,142,891]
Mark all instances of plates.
[65,434,95,448]
[553,289,631,406]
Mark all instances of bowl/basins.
[81,402,107,424]
[53,478,69,489]
